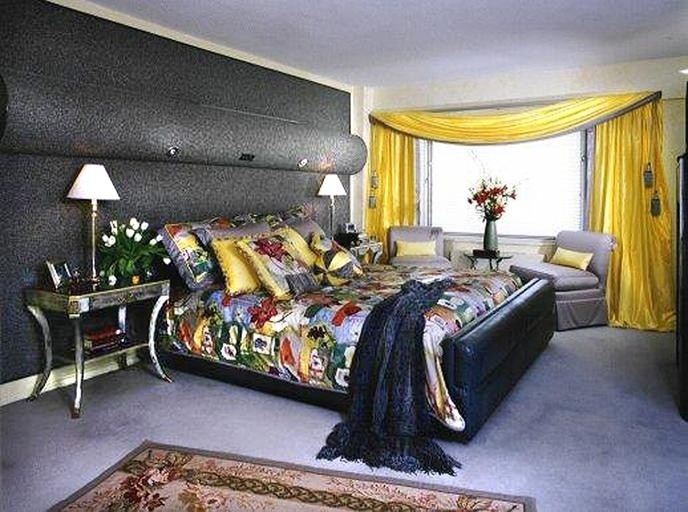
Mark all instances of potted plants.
[45,257,73,289]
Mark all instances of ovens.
[156,263,555,444]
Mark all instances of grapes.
[24,275,174,420]
[350,240,383,264]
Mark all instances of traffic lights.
[395,241,437,256]
[211,237,263,296]
[156,216,238,290]
[190,220,272,247]
[237,230,322,301]
[551,246,594,269]
[286,205,326,242]
[308,231,363,285]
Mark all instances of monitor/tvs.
[82,319,120,358]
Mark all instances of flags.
[484,218,498,250]
[136,262,161,280]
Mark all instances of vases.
[102,216,173,277]
[465,177,517,221]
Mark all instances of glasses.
[317,172,348,239]
[68,163,120,287]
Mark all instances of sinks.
[389,227,449,267]
[510,230,616,332]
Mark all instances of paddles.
[47,438,537,512]
